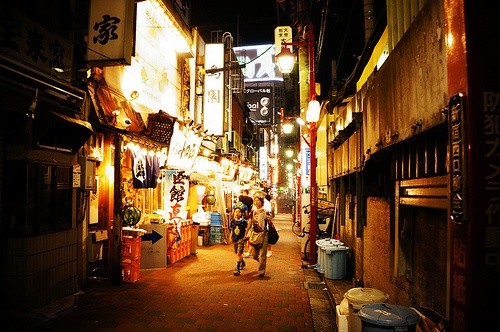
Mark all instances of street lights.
[273,19,320,267]
[283,116,302,232]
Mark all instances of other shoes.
[238,263,243,270]
[256,273,264,277]
[233,269,240,275]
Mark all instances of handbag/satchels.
[248,228,264,247]
[268,225,279,244]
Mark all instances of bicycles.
[299,204,328,264]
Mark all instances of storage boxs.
[120,236,141,283]
[336,306,349,332]
[209,212,223,244]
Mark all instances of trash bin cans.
[344,287,385,332]
[322,245,349,280]
[358,303,421,332]
[316,237,340,272]
[320,241,344,272]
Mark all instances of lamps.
[275,41,297,75]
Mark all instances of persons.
[244,196,271,276]
[239,195,275,257]
[230,207,248,275]
